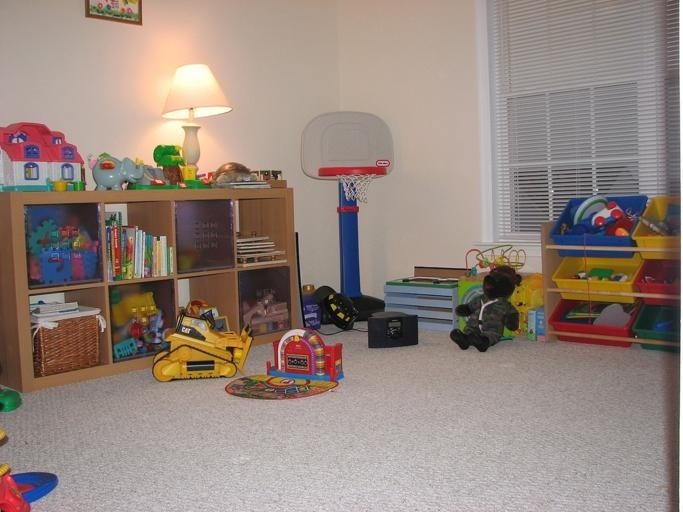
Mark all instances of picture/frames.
[83,1,145,27]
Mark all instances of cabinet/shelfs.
[538,218,680,352]
[0,181,302,397]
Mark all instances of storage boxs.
[456,265,534,341]
[380,273,457,331]
[545,189,680,352]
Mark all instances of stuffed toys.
[153,144,188,188]
[111,291,162,344]
[450,264,522,352]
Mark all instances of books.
[106,220,174,282]
[216,181,272,189]
[30,300,80,317]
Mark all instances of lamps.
[153,62,237,166]
